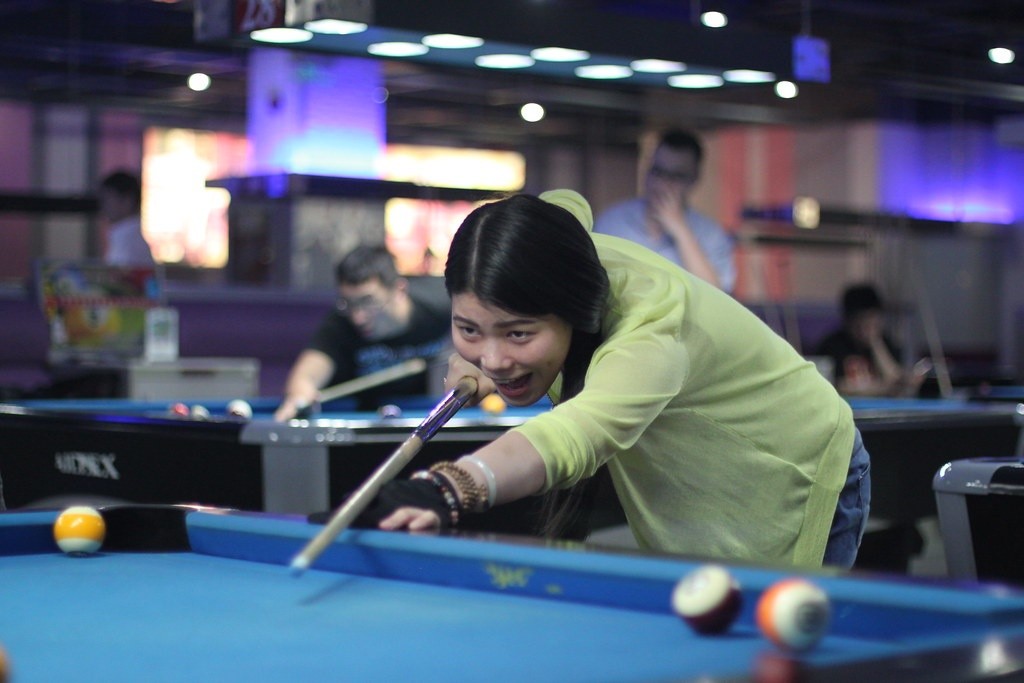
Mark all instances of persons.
[378,188,872,576]
[274,243,451,422]
[98,168,156,263]
[816,281,907,395]
[594,124,738,296]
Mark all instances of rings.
[443,376,448,383]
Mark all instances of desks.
[0,499,1024,683]
[0,395,1024,515]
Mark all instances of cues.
[318,356,424,404]
[290,376,480,578]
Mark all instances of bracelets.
[410,454,497,535]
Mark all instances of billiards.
[756,577,828,651]
[226,398,254,422]
[54,504,107,557]
[672,563,744,636]
[480,392,505,415]
[378,404,403,418]
[168,401,210,421]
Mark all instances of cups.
[143,305,180,364]
